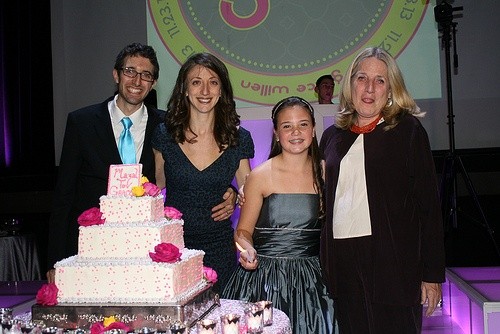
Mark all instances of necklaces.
[350,114,382,134]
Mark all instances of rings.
[226,211,229,215]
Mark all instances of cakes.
[36,163,218,305]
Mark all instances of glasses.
[120,66,155,83]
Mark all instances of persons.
[222,96,340,334]
[237,47,447,334]
[154,52,254,298]
[315,75,335,104]
[47,44,237,285]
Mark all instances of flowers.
[148,242,183,263]
[202,266,219,284]
[88,314,130,334]
[77,206,107,227]
[132,176,161,197]
[163,206,182,221]
[35,283,59,306]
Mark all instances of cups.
[243,306,264,334]
[256,299,274,326]
[170,324,188,334]
[220,312,240,334]
[0,308,166,334]
[197,319,217,334]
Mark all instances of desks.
[11,294,293,334]
[0,233,41,282]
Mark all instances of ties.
[118,117,136,166]
